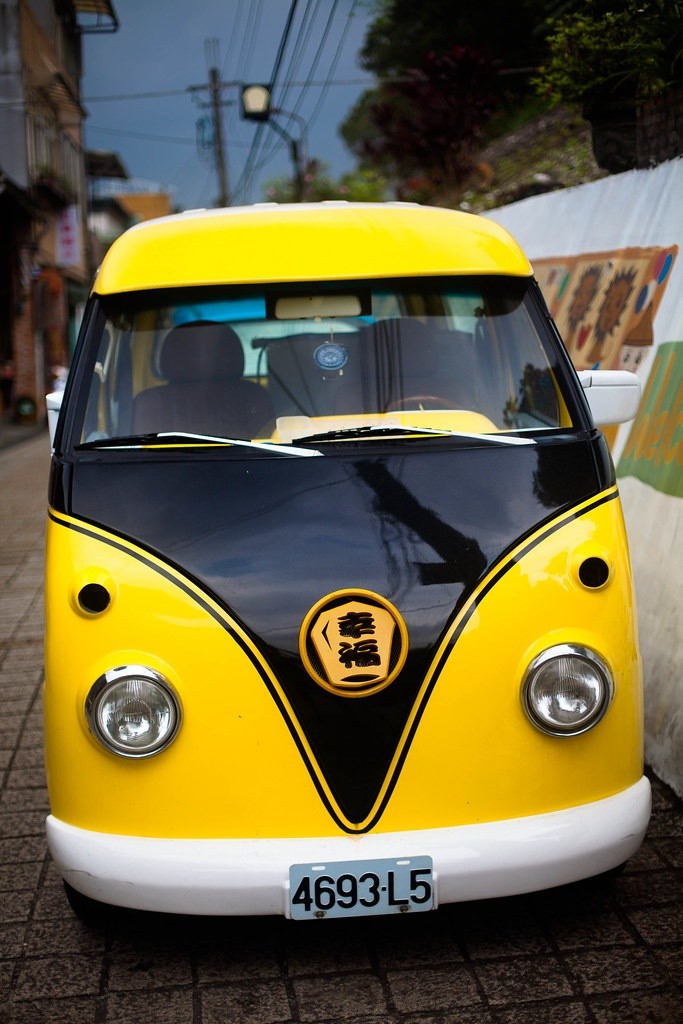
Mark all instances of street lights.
[239,80,314,202]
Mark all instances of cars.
[34,194,661,922]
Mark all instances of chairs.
[116,318,477,439]
